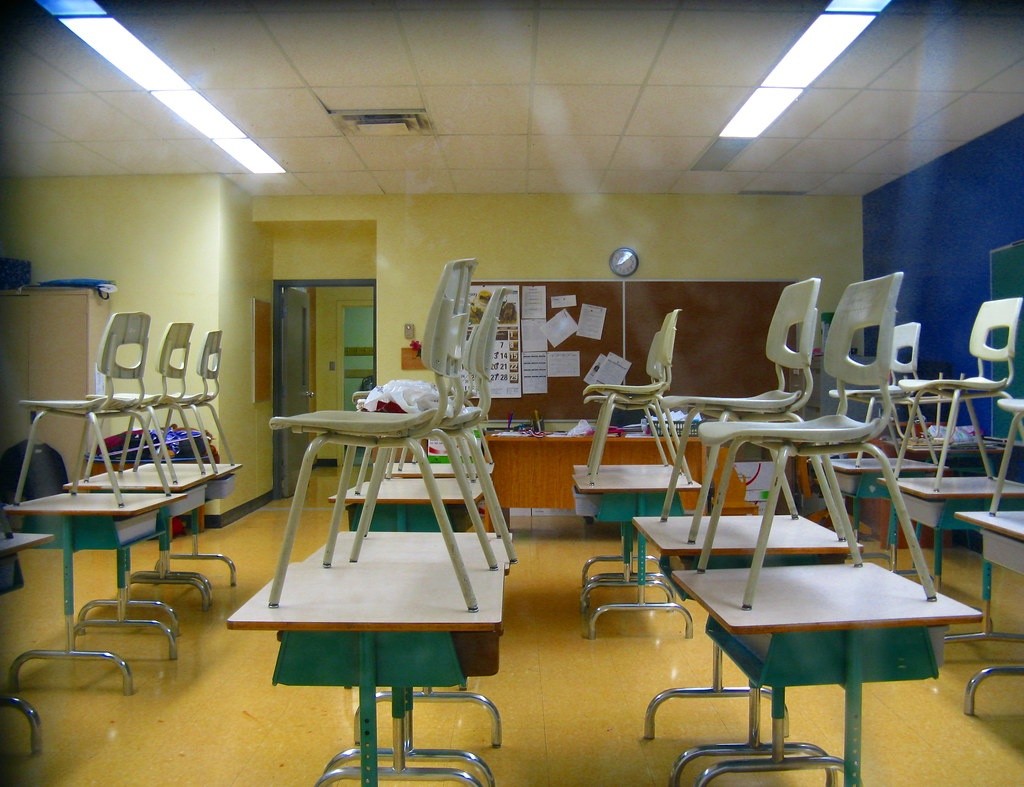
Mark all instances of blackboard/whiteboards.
[988,239,1024,447]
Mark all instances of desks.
[0,434,1023,787]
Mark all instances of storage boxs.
[706,439,775,501]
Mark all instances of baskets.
[649,420,701,438]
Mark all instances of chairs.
[15,272,1023,615]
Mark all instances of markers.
[534,409,542,432]
[506,410,515,430]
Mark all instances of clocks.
[609,247,640,277]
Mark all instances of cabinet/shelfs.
[0,287,114,485]
